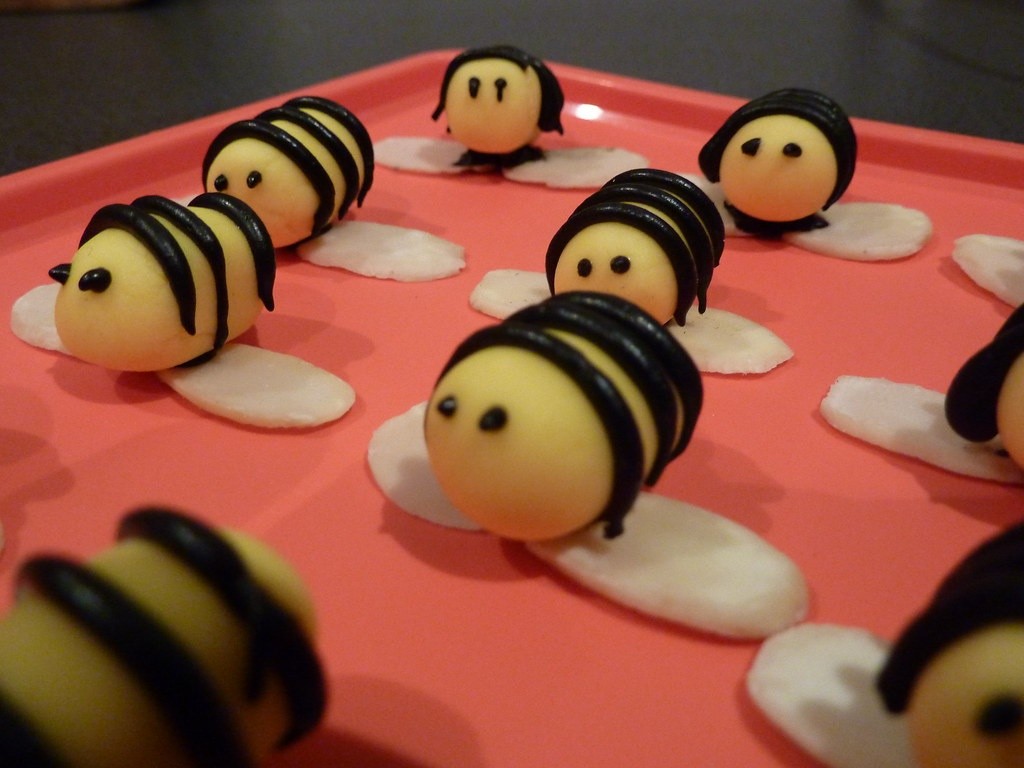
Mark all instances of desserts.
[0,42,1024,768]
